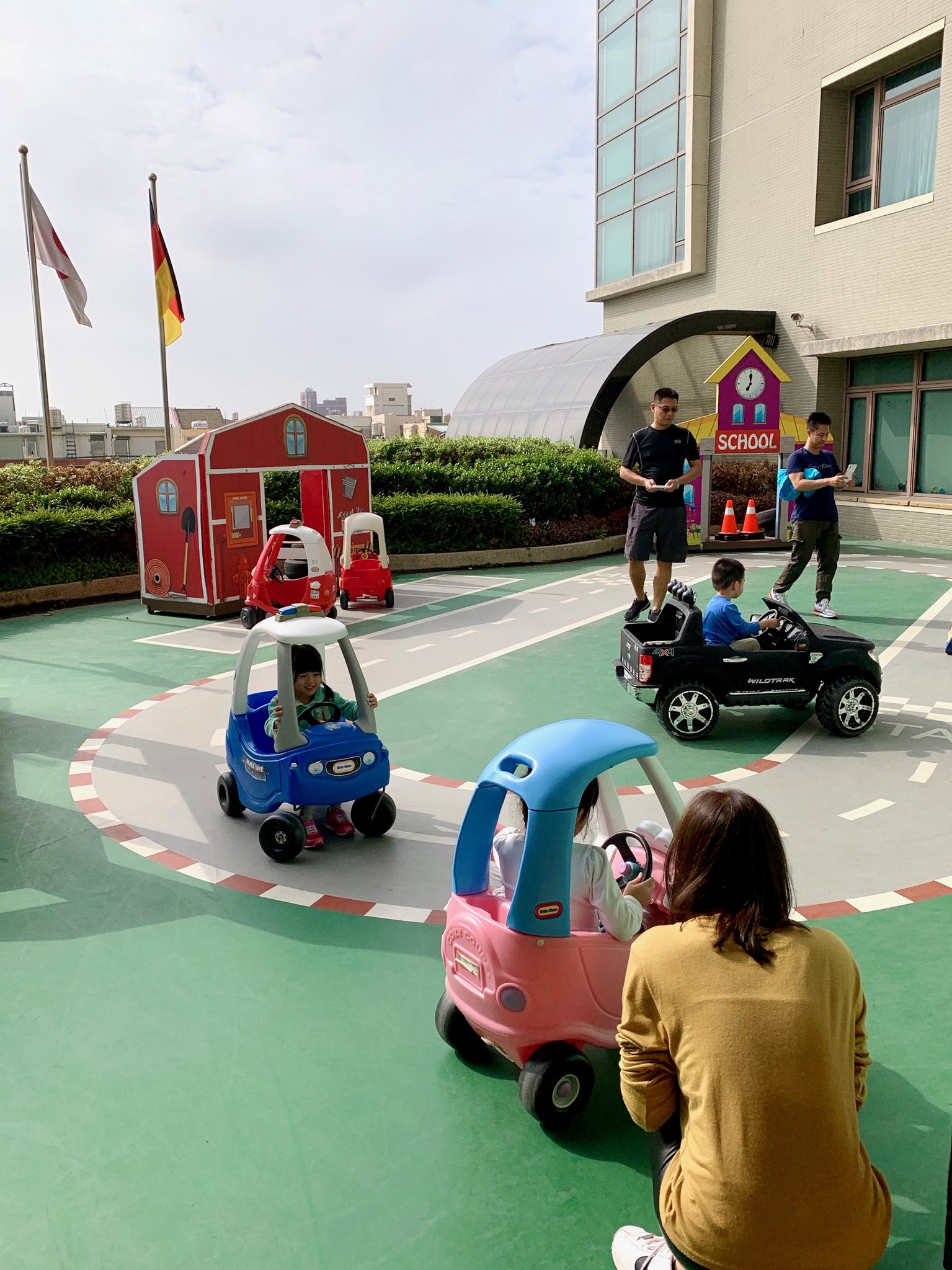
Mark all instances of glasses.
[655,404,679,412]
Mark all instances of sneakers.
[768,587,789,607]
[647,609,661,622]
[623,591,651,622]
[813,598,839,617]
[611,1225,676,1270]
[304,819,324,847]
[325,807,355,835]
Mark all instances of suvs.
[611,577,882,740]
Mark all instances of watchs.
[675,479,679,489]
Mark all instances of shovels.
[178,506,196,600]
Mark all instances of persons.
[620,387,702,623]
[702,557,780,650]
[264,644,378,850]
[945,618,952,655]
[769,411,856,618]
[493,766,655,942]
[611,790,892,1270]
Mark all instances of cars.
[239,519,339,630]
[434,718,686,1129]
[216,603,398,866]
[339,511,395,609]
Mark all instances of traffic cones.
[716,500,740,540]
[741,499,765,541]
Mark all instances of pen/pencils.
[343,506,363,520]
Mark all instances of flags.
[148,187,185,347]
[20,160,92,328]
